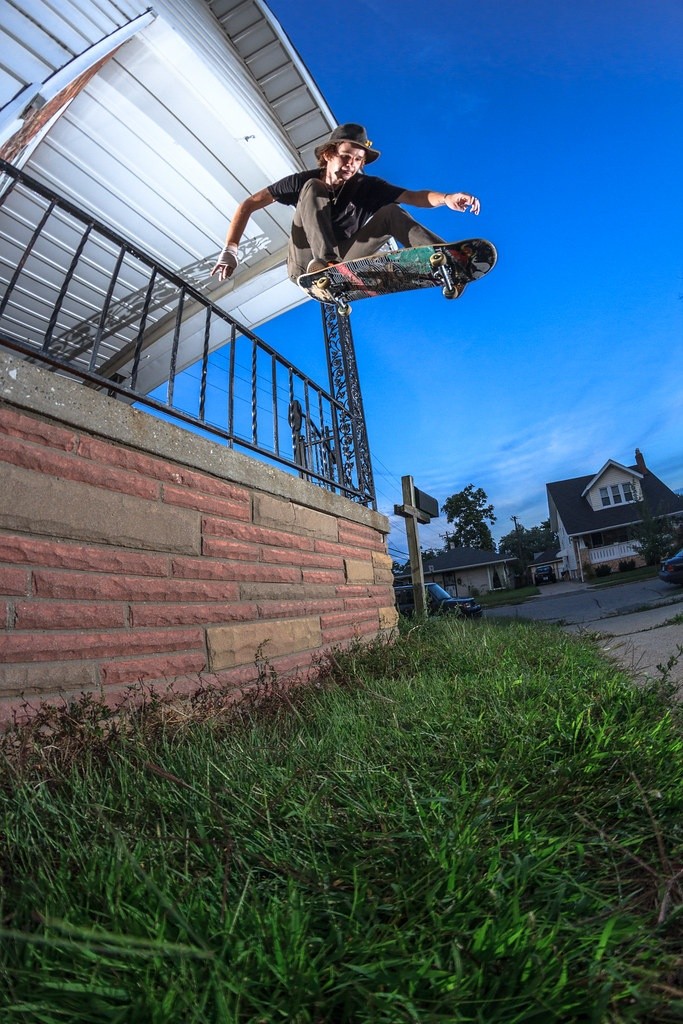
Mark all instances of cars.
[533,565,556,585]
[657,547,683,585]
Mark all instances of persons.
[211,125,480,287]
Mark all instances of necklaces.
[326,167,346,205]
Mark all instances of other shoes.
[306,256,329,275]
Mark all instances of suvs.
[394,582,483,621]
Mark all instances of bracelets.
[443,193,450,205]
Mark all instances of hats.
[314,123,381,165]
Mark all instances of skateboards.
[297,238,498,317]
